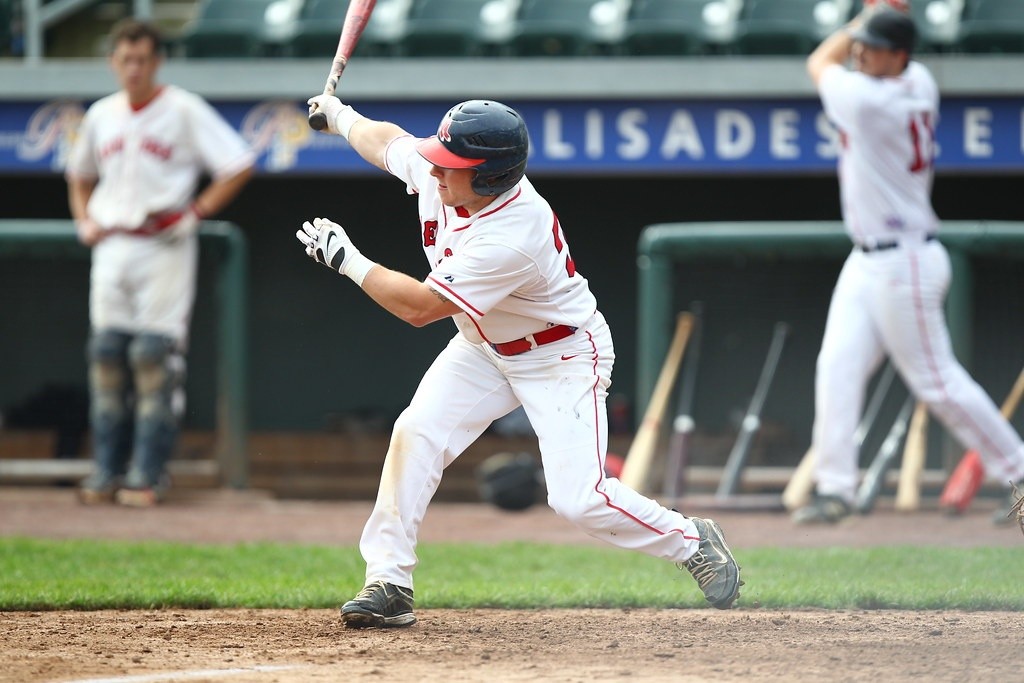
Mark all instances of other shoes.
[115,465,169,508]
[77,470,117,502]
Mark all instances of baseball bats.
[619,311,700,501]
[783,346,1024,514]
[308,0,377,132]
[646,492,783,510]
[719,322,790,496]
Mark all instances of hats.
[848,6,915,53]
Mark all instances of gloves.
[296,218,360,275]
[307,94,352,135]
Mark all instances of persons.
[62,18,257,507]
[785,0,1023,527]
[296,94,746,627]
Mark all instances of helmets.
[416,101,528,197]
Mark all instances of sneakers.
[992,478,1024,528]
[792,495,850,527]
[340,581,417,629]
[675,517,745,610]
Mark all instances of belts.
[862,233,935,252]
[487,308,597,356]
